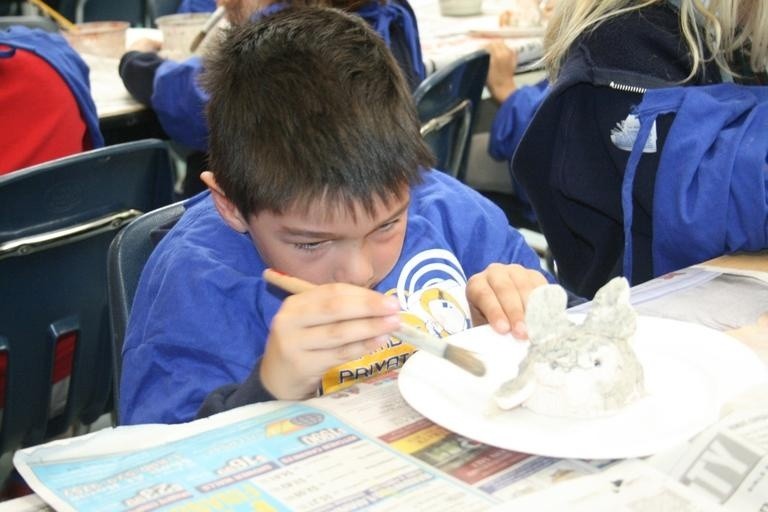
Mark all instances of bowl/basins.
[155,13,220,52]
[66,19,130,60]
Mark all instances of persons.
[0,23,105,178]
[111,8,592,428]
[509,0,768,309]
[467,42,560,160]
[118,0,429,193]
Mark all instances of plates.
[397,314,767,459]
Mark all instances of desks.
[2,238,767,512]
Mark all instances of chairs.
[102,189,229,428]
[2,133,198,502]
[405,49,495,189]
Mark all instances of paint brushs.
[190,6,229,51]
[262,269,487,375]
